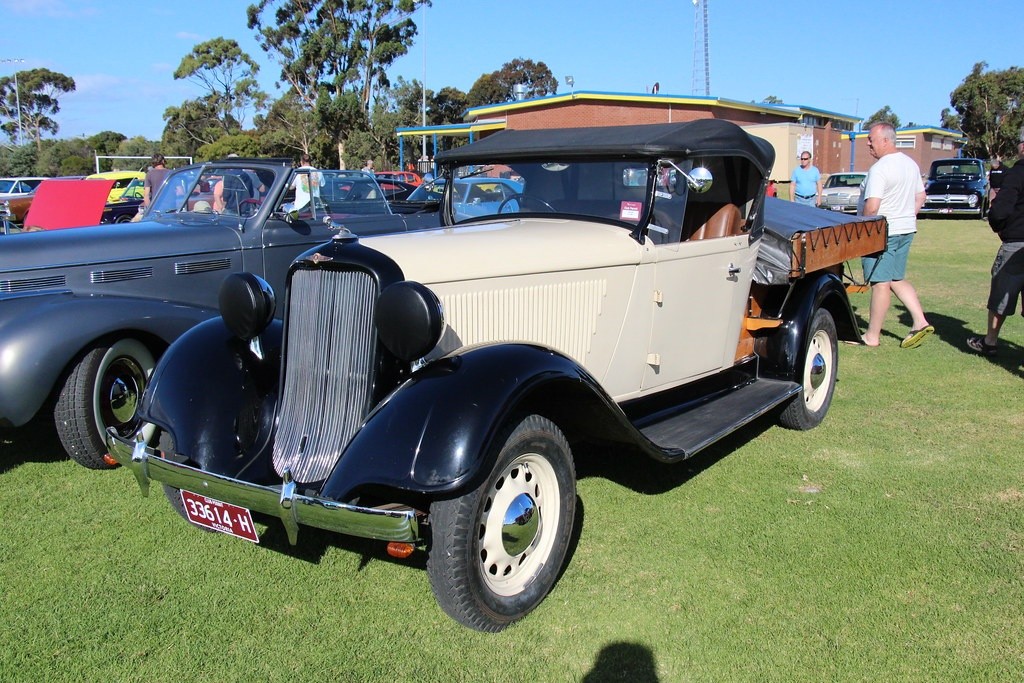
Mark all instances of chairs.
[836,180,847,186]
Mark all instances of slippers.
[901,326,934,349]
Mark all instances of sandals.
[967,336,999,357]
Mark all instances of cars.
[105,118,892,634]
[917,157,990,217]
[0,152,455,469]
[0,170,221,231]
[821,172,867,211]
[373,171,421,189]
[320,175,441,200]
[405,175,524,225]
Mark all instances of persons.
[986,158,1009,215]
[361,160,376,200]
[967,126,1024,354]
[405,159,423,180]
[212,153,266,218]
[289,155,327,212]
[789,151,823,209]
[845,123,936,348]
[130,153,185,222]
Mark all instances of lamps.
[565,75,575,99]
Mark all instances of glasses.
[801,158,808,160]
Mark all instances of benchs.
[548,199,740,243]
[472,192,504,202]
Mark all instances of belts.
[795,194,815,199]
